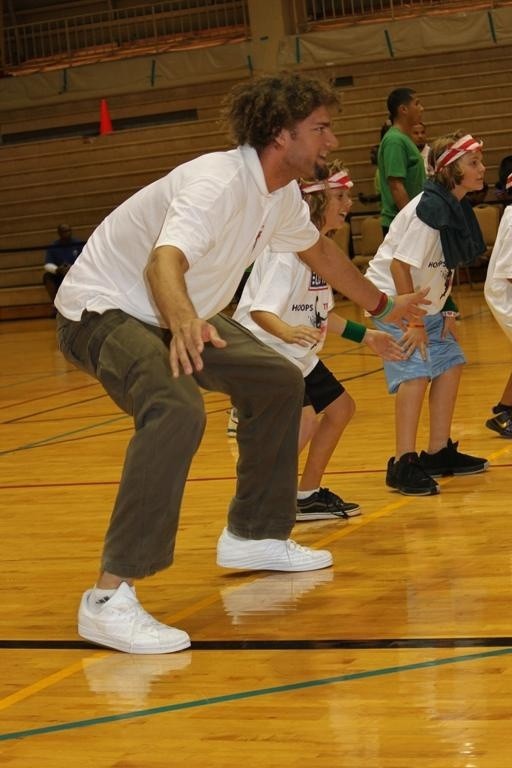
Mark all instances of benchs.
[301,52,509,255]
[0,50,256,312]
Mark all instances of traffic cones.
[97,97,113,135]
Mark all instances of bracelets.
[441,310,460,318]
[406,323,424,329]
[366,292,389,317]
[374,297,395,322]
[342,318,366,343]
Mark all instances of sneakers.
[80,652,193,703]
[212,526,334,575]
[225,408,239,438]
[293,488,362,520]
[385,451,439,496]
[75,583,193,658]
[220,570,334,624]
[419,438,490,477]
[486,405,512,437]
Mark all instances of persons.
[42,221,90,320]
[52,70,435,656]
[230,157,409,523]
[360,126,489,499]
[409,121,435,182]
[372,121,391,198]
[481,156,511,438]
[376,87,430,240]
